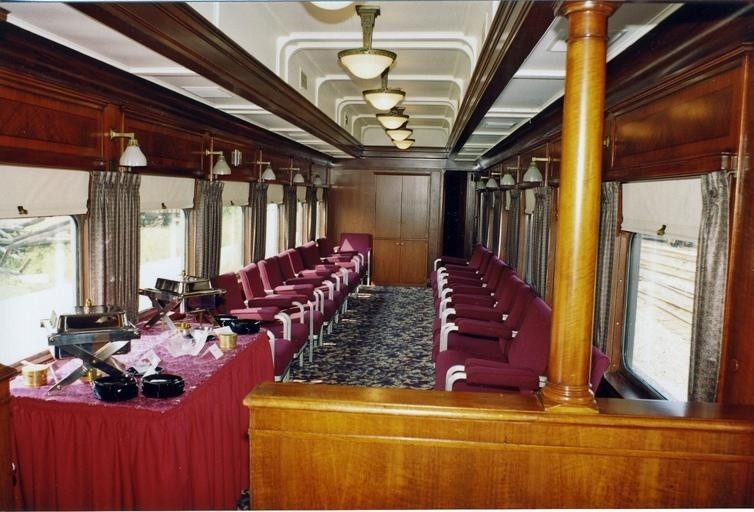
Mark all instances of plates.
[216,315,260,334]
[93,374,185,400]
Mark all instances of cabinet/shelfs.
[372,172,430,286]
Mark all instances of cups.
[220,334,237,350]
[22,364,46,387]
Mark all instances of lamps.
[204,150,324,187]
[475,157,551,191]
[336,5,415,150]
[107,128,150,169]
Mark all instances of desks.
[10,318,271,509]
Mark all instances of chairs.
[208,231,373,383]
[431,244,612,397]
